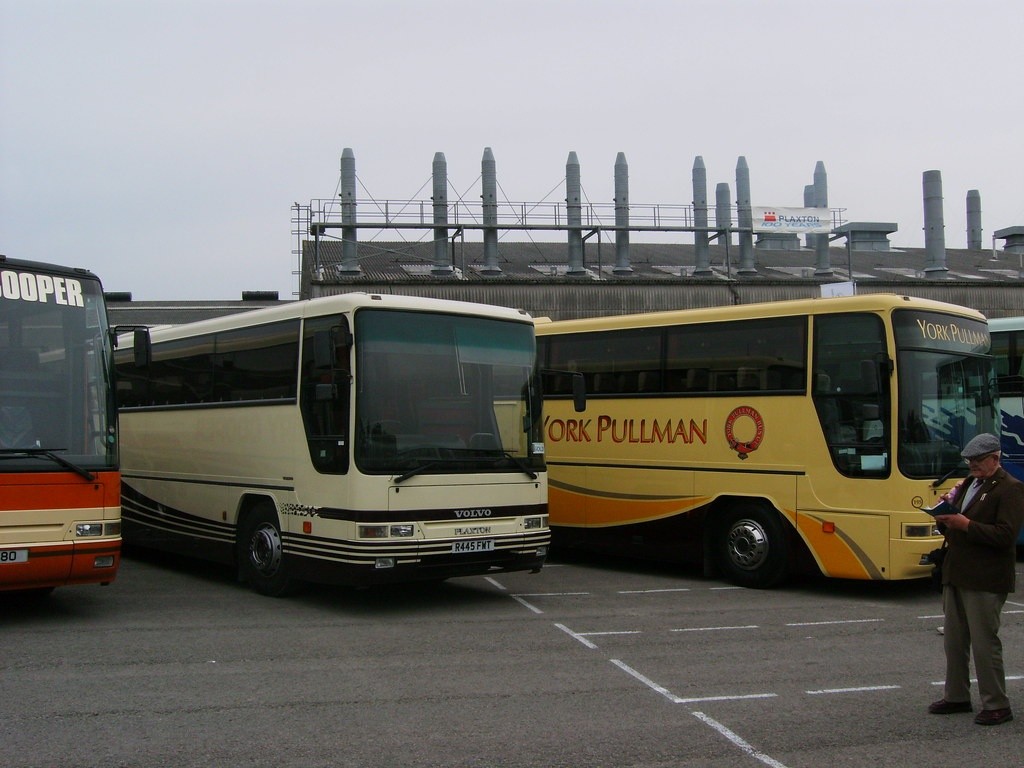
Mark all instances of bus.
[473,295,993,589]
[42,296,586,595]
[0,254,152,594]
[915,316,1023,557]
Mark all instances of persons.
[928,433,1024,726]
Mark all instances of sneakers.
[928,699,972,714]
[975,706,1013,725]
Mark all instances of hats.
[961,434,1001,457]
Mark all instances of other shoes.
[936,626,944,634]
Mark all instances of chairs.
[829,368,850,396]
[638,372,660,393]
[815,374,850,437]
[765,365,802,390]
[737,367,766,390]
[709,372,736,391]
[618,375,637,393]
[687,369,710,392]
[554,375,571,394]
[594,374,619,394]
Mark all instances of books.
[920,500,958,517]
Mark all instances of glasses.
[964,453,993,466]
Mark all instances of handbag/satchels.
[929,545,947,591]
